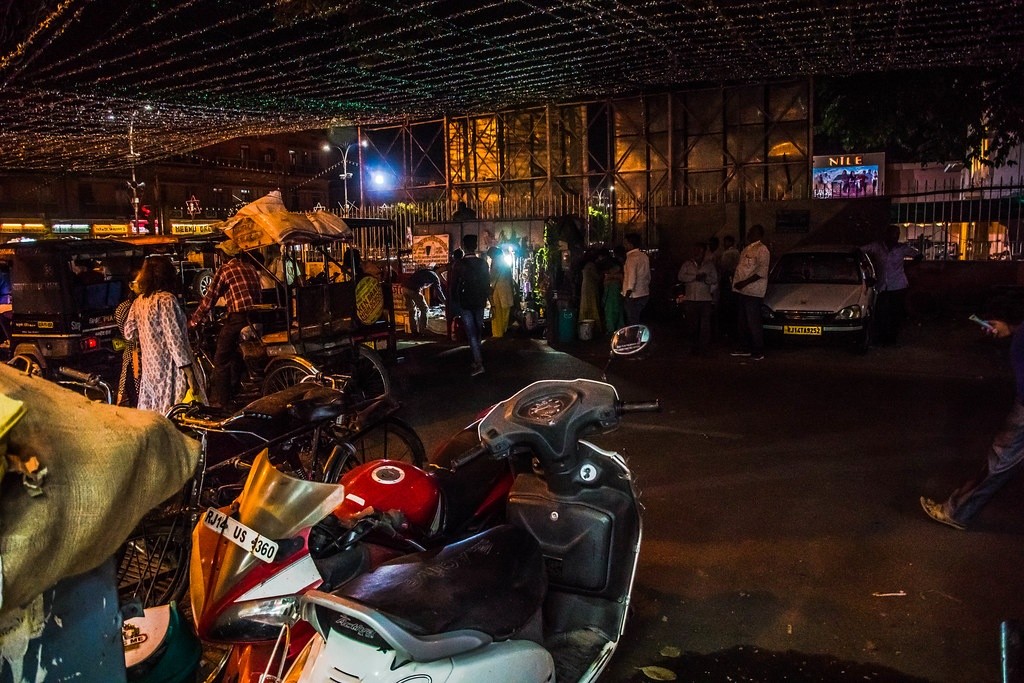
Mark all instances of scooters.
[263,324,664,682]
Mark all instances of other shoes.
[730,350,751,356]
[749,354,768,359]
[920,495,968,532]
[470,365,485,376]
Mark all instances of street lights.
[108,103,151,219]
[320,139,364,225]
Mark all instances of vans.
[10,238,152,368]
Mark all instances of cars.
[772,251,876,343]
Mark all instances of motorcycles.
[168,421,691,683]
[161,355,431,473]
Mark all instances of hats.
[214,240,239,257]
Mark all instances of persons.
[818,169,878,195]
[340,237,361,272]
[860,226,923,343]
[731,224,771,360]
[679,242,718,356]
[115,264,142,408]
[402,265,446,339]
[487,247,514,341]
[0,261,12,304]
[445,248,463,340]
[920,320,1024,531]
[188,240,262,408]
[452,201,476,219]
[620,233,651,326]
[707,237,722,262]
[268,245,301,308]
[123,255,209,415]
[720,235,741,283]
[453,234,489,377]
[74,254,104,285]
[545,240,626,345]
